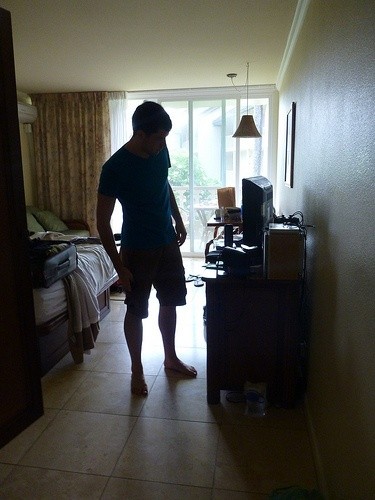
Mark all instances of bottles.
[243,387,265,417]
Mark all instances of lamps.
[227,63,263,139]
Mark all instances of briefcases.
[28,230,78,289]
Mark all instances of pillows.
[32,211,69,231]
[27,211,44,232]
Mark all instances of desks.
[201,260,307,405]
[206,213,243,247]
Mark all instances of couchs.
[26,207,91,236]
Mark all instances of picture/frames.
[281,102,294,188]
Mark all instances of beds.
[33,243,120,379]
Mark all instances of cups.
[215,209,221,218]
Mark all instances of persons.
[97,100,197,397]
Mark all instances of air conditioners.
[17,101,39,123]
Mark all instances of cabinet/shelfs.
[0,7,44,449]
